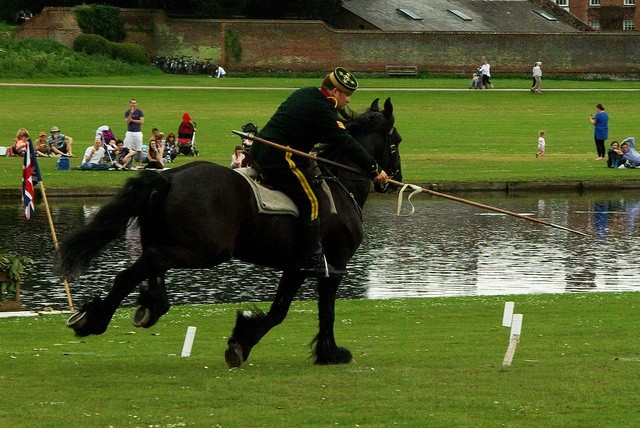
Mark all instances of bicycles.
[145,55,215,75]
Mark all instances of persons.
[480,56,488,66]
[231,146,245,169]
[620,137,640,168]
[134,145,149,163]
[108,140,116,147]
[124,99,144,169]
[163,133,177,162]
[607,141,626,169]
[111,140,137,171]
[532,62,537,87]
[149,128,165,142]
[72,138,109,170]
[47,126,74,158]
[16,135,29,156]
[531,62,543,94]
[33,132,62,158]
[589,104,609,160]
[251,66,389,278]
[469,73,479,89]
[143,140,163,169]
[536,130,545,158]
[214,65,226,79]
[15,128,29,141]
[478,60,494,89]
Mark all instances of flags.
[20,137,42,221]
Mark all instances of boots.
[304,217,348,275]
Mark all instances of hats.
[328,67,358,95]
[51,126,60,131]
[40,132,46,136]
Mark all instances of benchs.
[385,66,418,78]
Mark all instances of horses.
[51,97,404,367]
